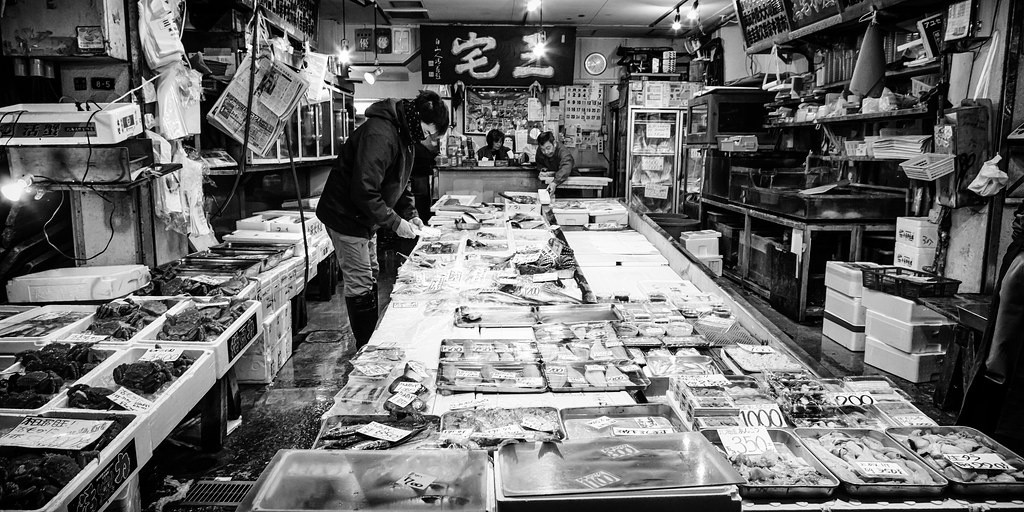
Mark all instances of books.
[814,13,946,86]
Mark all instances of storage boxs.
[1,211,339,512]
[680,229,723,276]
[821,215,966,383]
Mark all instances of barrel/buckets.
[652,218,702,243]
[645,211,689,219]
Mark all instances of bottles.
[469,149,475,158]
[458,149,463,166]
[451,148,456,167]
[467,137,472,150]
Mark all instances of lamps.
[364,6,384,84]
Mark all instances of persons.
[315,89,450,346]
[475,129,514,159]
[536,130,583,198]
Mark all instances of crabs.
[137,259,249,298]
[83,294,168,341]
[156,296,250,341]
[0,446,101,510]
[64,355,196,410]
[0,339,108,410]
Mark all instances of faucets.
[815,122,840,150]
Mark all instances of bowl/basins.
[457,224,482,229]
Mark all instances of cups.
[508,159,515,166]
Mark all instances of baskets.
[860,265,962,298]
[898,152,956,181]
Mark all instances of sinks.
[751,186,906,219]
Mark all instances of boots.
[346,284,378,352]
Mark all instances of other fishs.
[301,192,1024,508]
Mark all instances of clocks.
[584,52,607,75]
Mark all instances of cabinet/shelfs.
[762,57,946,162]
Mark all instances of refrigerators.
[615,80,706,213]
